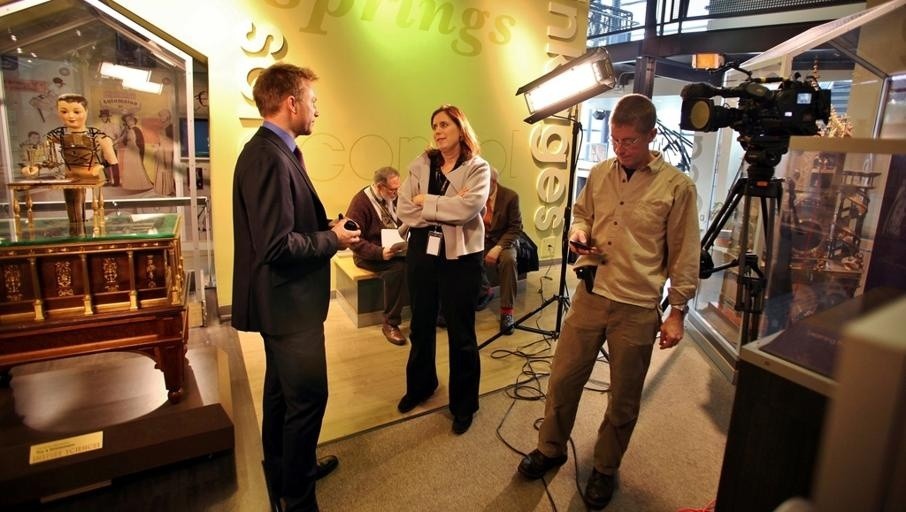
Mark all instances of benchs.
[334,255,527,329]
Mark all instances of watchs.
[671,304,689,314]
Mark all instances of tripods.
[477,104,611,365]
[662,177,794,346]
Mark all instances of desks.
[5,176,109,235]
[1,269,193,431]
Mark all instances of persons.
[111,114,154,191]
[343,166,411,345]
[517,93,702,510]
[396,104,490,438]
[231,65,363,512]
[45,94,119,177]
[479,167,522,336]
[98,109,122,187]
[153,109,176,195]
[18,131,44,162]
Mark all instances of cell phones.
[569,240,593,250]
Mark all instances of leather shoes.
[381,321,407,346]
[399,391,419,413]
[451,414,475,435]
[313,454,338,480]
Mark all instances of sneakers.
[584,467,616,507]
[500,312,514,335]
[475,288,495,311]
[518,448,568,479]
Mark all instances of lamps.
[97,58,152,83]
[122,80,164,95]
[515,46,617,124]
